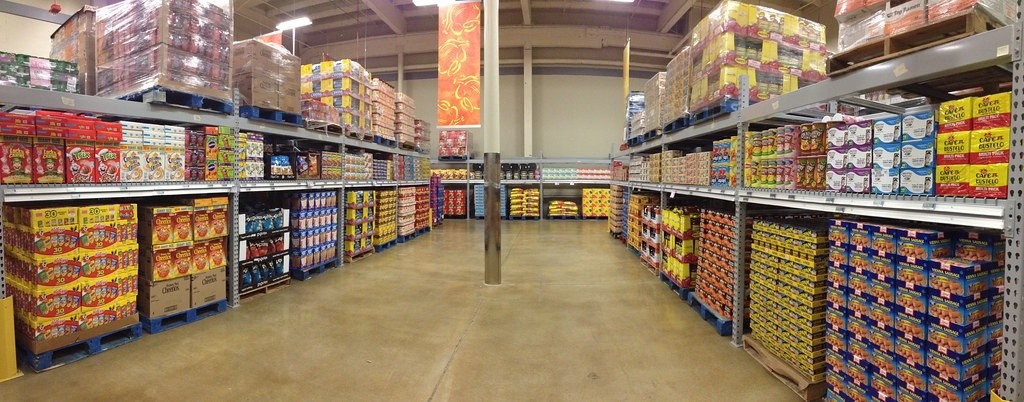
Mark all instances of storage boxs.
[0,0,465,355]
[474,0,1024,402]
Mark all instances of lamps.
[412,0,456,8]
[275,17,312,30]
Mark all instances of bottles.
[642,205,700,286]
